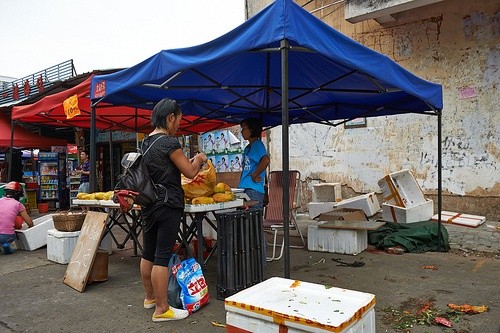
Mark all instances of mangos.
[192,183,236,204]
[77,191,114,200]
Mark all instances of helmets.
[4,181,22,192]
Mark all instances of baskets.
[53,208,87,231]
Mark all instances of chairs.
[265,170,305,262]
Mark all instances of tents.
[89,0,444,279]
[7,74,240,192]
[0,109,67,180]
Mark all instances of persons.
[237,118,270,209]
[74,149,90,210]
[141,98,207,322]
[0,181,33,254]
[204,132,240,171]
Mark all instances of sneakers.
[152,305,189,322]
[143,297,156,308]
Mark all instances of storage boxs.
[224,277,375,333]
[15,214,112,264]
[307,169,434,255]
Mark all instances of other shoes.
[3,243,14,255]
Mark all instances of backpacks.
[117,134,168,209]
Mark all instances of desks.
[72,198,244,270]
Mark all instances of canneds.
[40,169,58,189]
[71,173,81,197]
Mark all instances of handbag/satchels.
[167,254,183,308]
